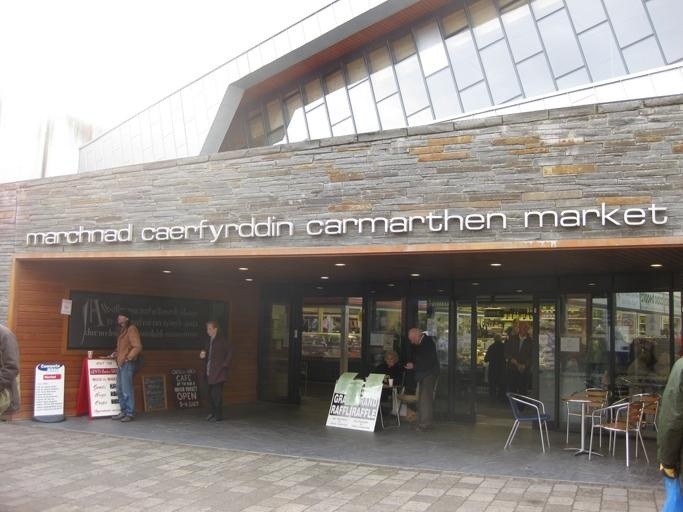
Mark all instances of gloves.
[659,463,678,479]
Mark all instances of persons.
[654,353,682,512]
[401,327,441,433]
[108,310,144,423]
[0,324,20,415]
[504,318,520,340]
[196,318,235,423]
[505,319,534,412]
[483,333,511,409]
[370,350,403,387]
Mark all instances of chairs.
[588,400,649,467]
[396,375,440,426]
[503,391,552,454]
[566,387,612,449]
[609,392,661,457]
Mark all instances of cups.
[388,378,394,386]
[87,350,93,359]
[200,350,206,359]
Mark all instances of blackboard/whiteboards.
[142,375,167,413]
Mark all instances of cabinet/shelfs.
[298,293,682,367]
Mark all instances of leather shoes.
[112,414,125,419]
[208,414,224,421]
[121,416,132,421]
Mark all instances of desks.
[376,383,404,430]
[562,397,605,457]
